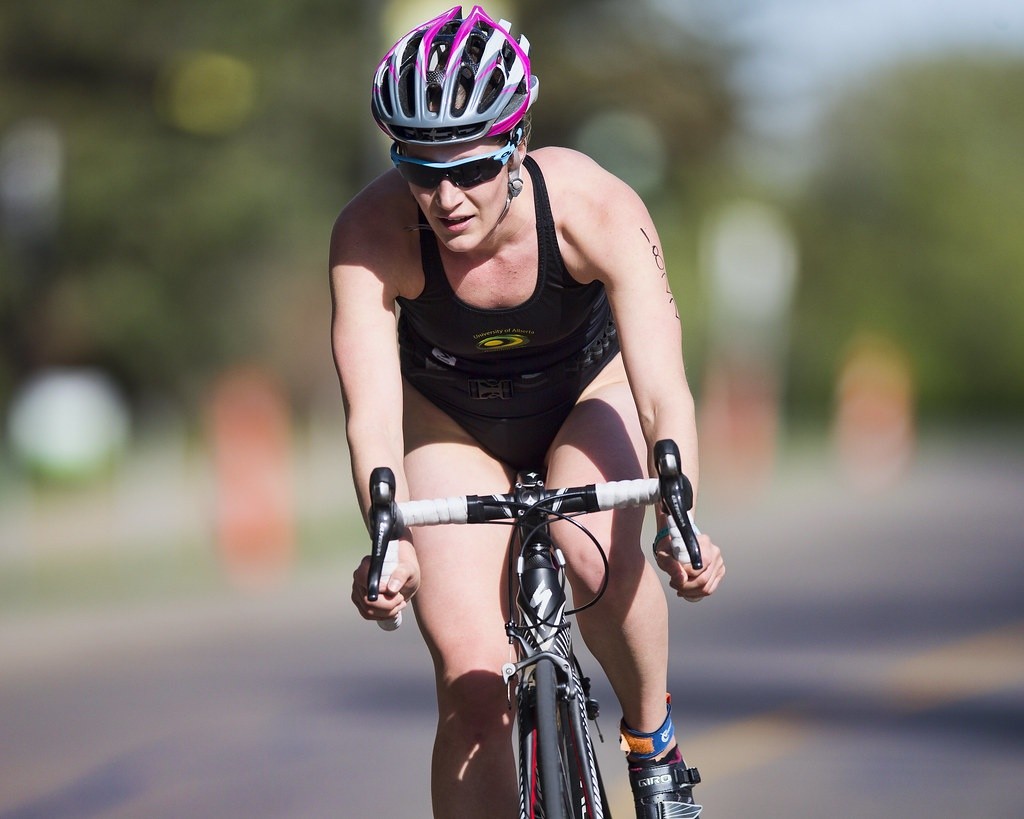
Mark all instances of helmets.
[371,5,539,145]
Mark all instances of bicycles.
[368,437,702,819]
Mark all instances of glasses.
[390,127,522,190]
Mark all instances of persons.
[330,5,725,819]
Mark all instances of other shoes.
[628,743,701,819]
